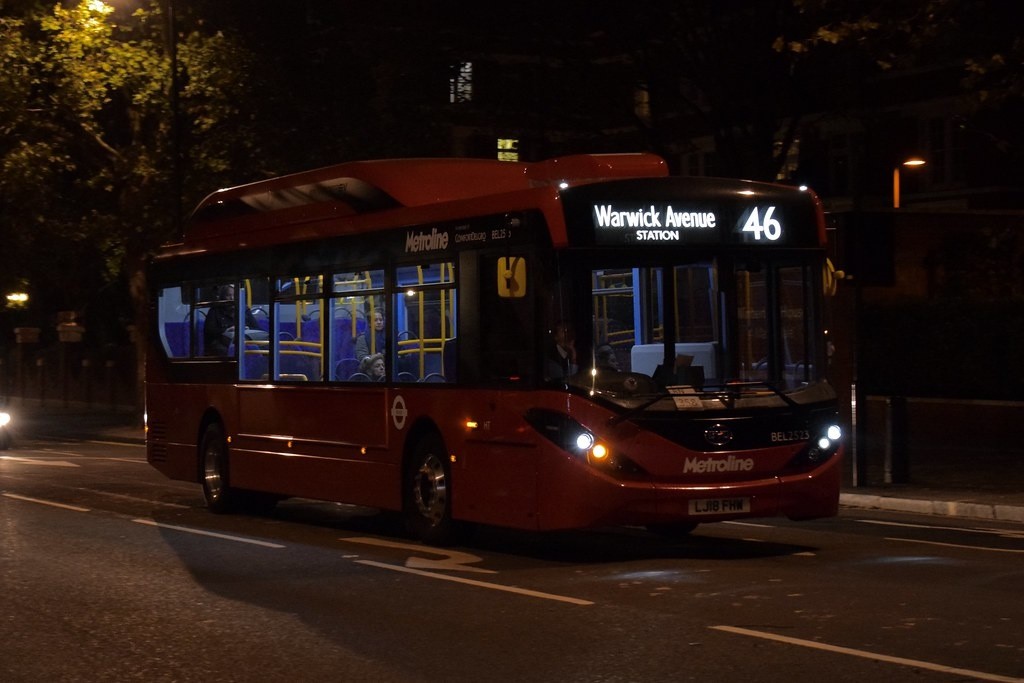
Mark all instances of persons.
[356,352,386,383]
[200,284,263,357]
[596,343,622,372]
[543,320,579,382]
[354,306,386,361]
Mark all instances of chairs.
[182,308,446,382]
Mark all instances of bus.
[141,151,846,552]
